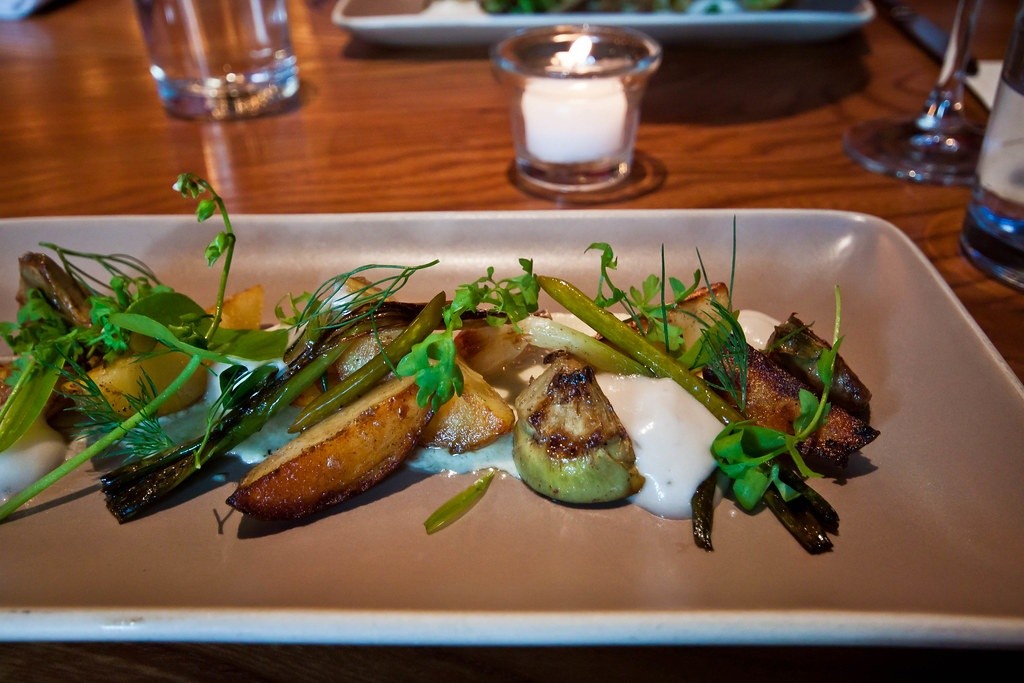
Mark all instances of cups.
[960,9,1024,290]
[492,24,661,192]
[134,0,300,118]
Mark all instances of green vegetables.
[0,172,847,554]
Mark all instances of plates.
[0,209,1024,649]
[328,0,875,43]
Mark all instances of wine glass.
[841,0,986,185]
